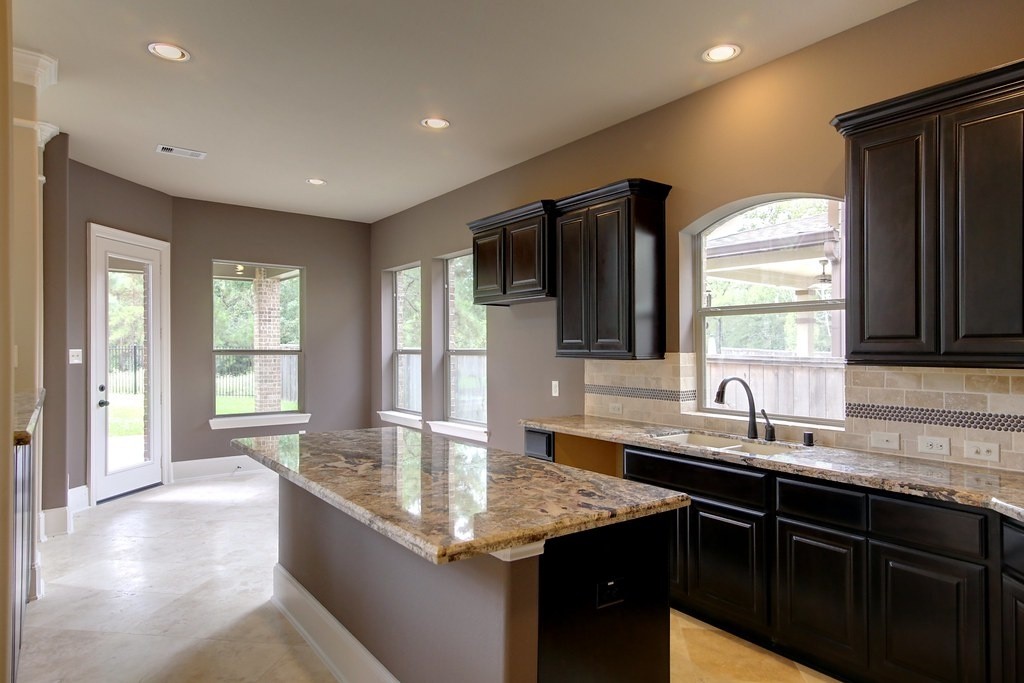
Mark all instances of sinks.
[653,432,742,452]
[726,442,794,455]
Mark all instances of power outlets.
[964,441,1000,463]
[870,431,900,450]
[917,435,951,455]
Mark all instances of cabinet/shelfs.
[466,200,555,309]
[548,175,673,361]
[827,56,1024,369]
[524,427,1024,682]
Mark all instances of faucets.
[714,375,759,438]
